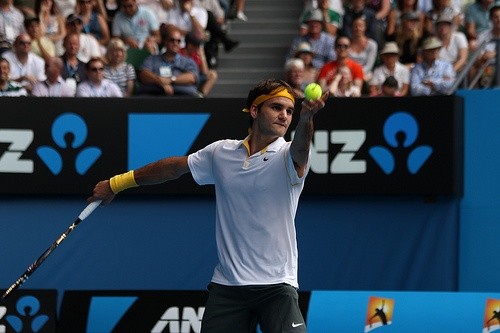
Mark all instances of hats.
[294,42,314,57]
[435,14,453,25]
[66,14,83,25]
[401,14,419,22]
[380,42,400,54]
[304,10,327,25]
[422,37,442,50]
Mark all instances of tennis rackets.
[3,199,102,299]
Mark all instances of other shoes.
[226,41,239,52]
[237,12,248,24]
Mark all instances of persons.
[87,78,328,333]
[0,0,500,97]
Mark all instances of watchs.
[170,76,176,84]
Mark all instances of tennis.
[303,83,321,101]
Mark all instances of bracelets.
[109,171,138,194]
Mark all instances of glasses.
[336,45,349,49]
[168,38,181,44]
